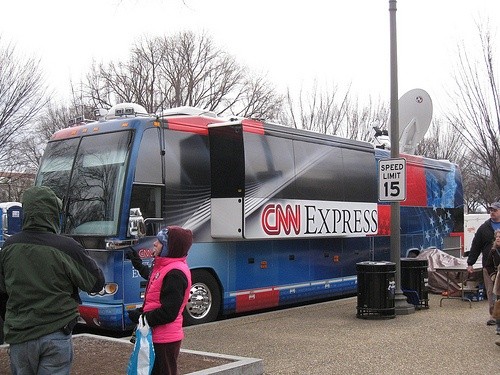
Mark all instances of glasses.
[495,236,500,238]
[489,209,497,213]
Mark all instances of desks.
[435,264,484,309]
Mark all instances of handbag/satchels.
[493,300,500,320]
[126,314,156,375]
[492,266,500,296]
[489,272,496,281]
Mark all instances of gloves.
[125,245,146,274]
[126,308,148,326]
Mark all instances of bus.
[34,103,465,332]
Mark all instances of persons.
[124,226,194,375]
[0,185,104,375]
[466,202,500,346]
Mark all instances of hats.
[488,202,500,209]
[156,228,168,257]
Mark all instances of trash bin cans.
[355,261,397,321]
[400,257,430,311]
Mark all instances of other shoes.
[487,319,496,325]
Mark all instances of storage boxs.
[464,283,484,302]
